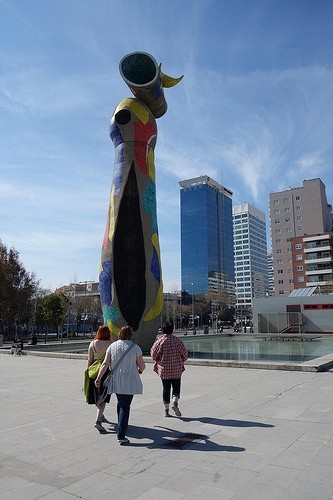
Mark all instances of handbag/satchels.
[104,374,111,387]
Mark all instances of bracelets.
[138,368,142,374]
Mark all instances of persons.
[87,324,112,433]
[28,333,38,345]
[9,336,23,356]
[150,325,189,417]
[94,326,146,445]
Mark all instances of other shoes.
[93,421,108,435]
[100,414,108,423]
[163,409,172,417]
[118,439,131,446]
[172,405,183,417]
